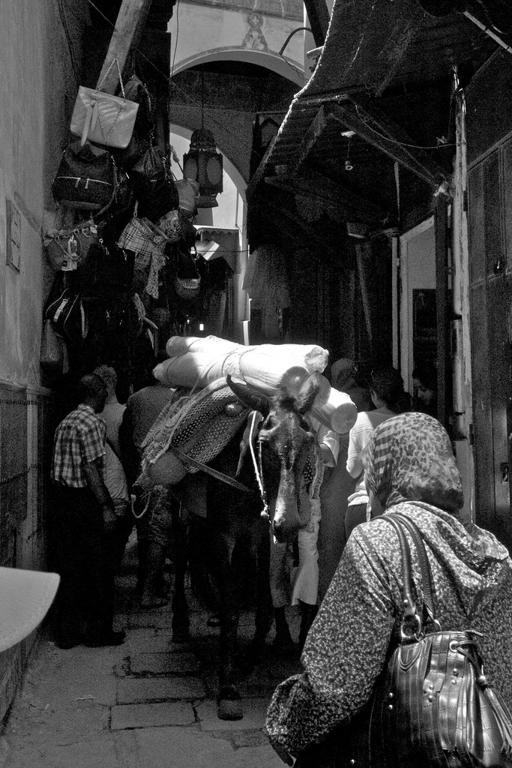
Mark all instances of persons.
[46,356,438,653]
[265,409,511,768]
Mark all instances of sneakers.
[56,630,125,649]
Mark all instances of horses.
[118,376,320,719]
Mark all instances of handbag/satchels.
[294,630,512,766]
